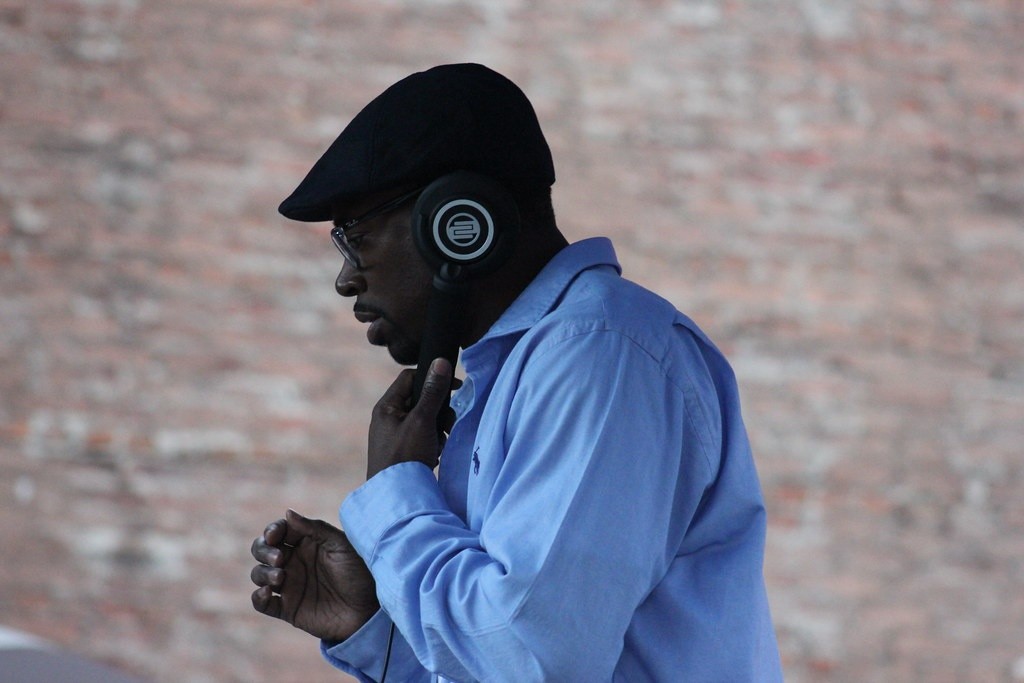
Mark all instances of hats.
[280,63,554,222]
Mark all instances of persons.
[249,62,782,683]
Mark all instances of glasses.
[330,185,426,268]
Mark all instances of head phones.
[407,168,524,434]
